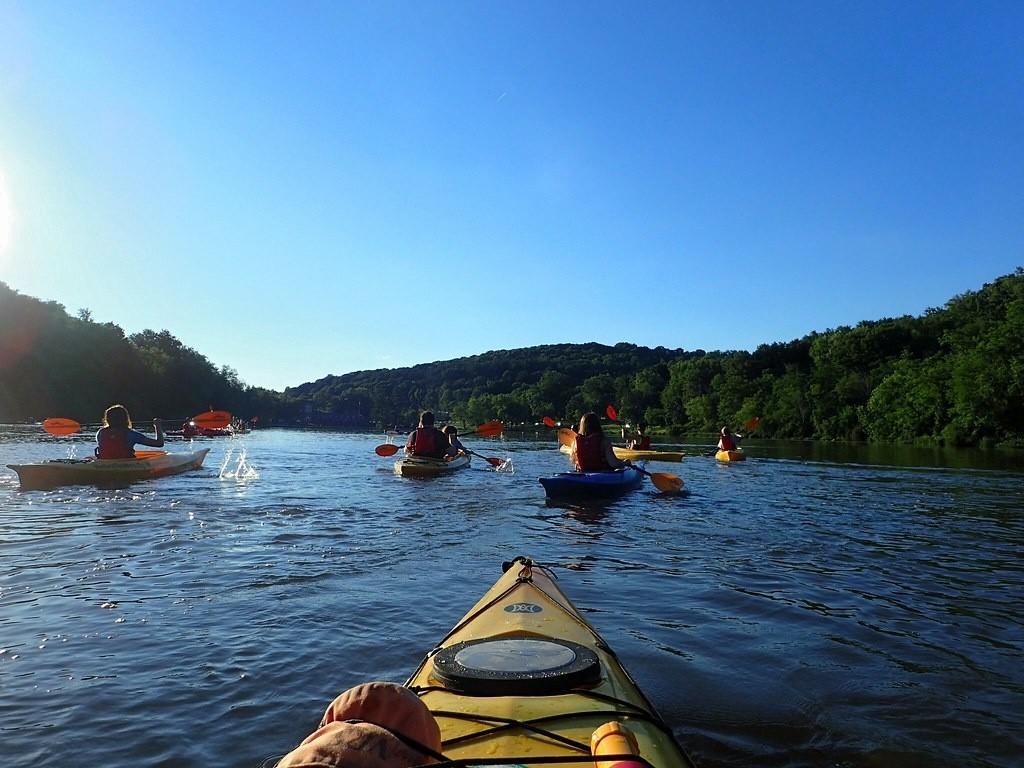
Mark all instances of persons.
[569,413,631,472]
[183,416,198,433]
[620,423,651,451]
[718,427,742,451]
[404,410,474,460]
[95,405,164,459]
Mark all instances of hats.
[721,427,729,434]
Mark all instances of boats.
[537,459,645,500]
[393,449,472,477]
[224,427,251,433]
[715,447,746,463]
[164,428,230,436]
[559,442,688,464]
[6,447,211,491]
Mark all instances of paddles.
[606,406,624,429]
[706,416,759,455]
[473,453,505,466]
[556,428,685,492]
[42,410,232,436]
[375,422,503,457]
[543,417,560,429]
[386,430,410,435]
